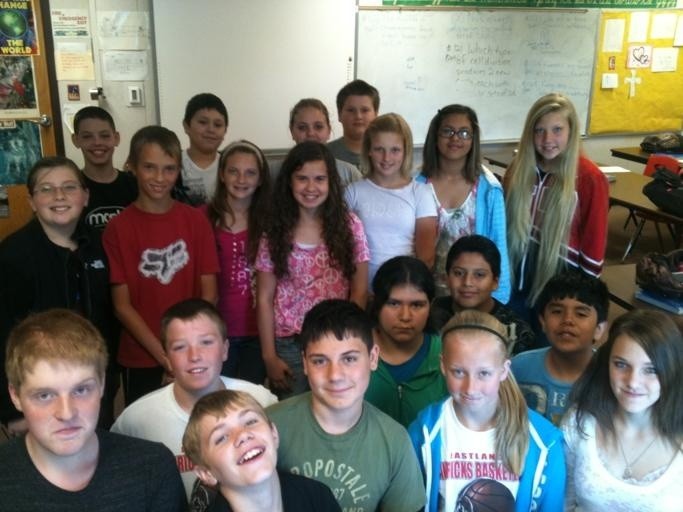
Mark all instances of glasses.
[438,126,473,141]
[32,182,82,194]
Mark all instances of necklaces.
[604,415,661,480]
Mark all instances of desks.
[482,151,683,263]
[612,147,683,170]
[599,265,683,329]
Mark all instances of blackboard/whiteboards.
[353,7,600,149]
[150,0,384,158]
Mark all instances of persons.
[264,98,363,193]
[0,156,121,440]
[363,256,451,432]
[422,234,536,357]
[69,106,138,230]
[101,125,222,411]
[111,297,278,505]
[182,389,341,512]
[412,104,514,306]
[254,140,372,403]
[195,139,271,385]
[502,91,609,318]
[178,92,229,203]
[0,308,189,512]
[328,78,381,179]
[406,310,567,512]
[342,113,438,307]
[262,298,429,512]
[559,309,683,511]
[509,268,610,429]
